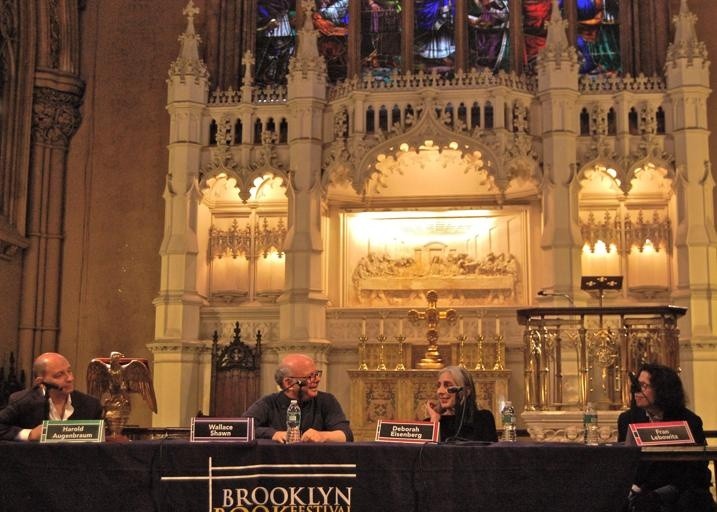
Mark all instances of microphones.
[444,387,471,444]
[296,380,308,408]
[628,372,641,424]
[40,381,63,423]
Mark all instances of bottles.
[502,399,517,443]
[584,403,598,446]
[287,399,301,443]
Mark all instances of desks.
[2,441,704,512]
[516,305,689,410]
